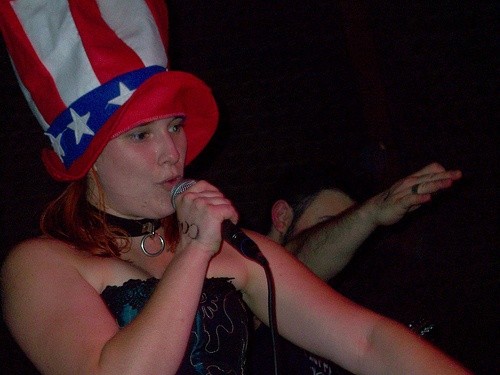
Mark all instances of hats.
[1,0,218,181]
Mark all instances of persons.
[1,1,479,374]
[235,165,463,374]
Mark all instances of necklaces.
[84,199,167,257]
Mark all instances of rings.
[411,183,422,193]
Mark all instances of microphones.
[171,179,270,269]
